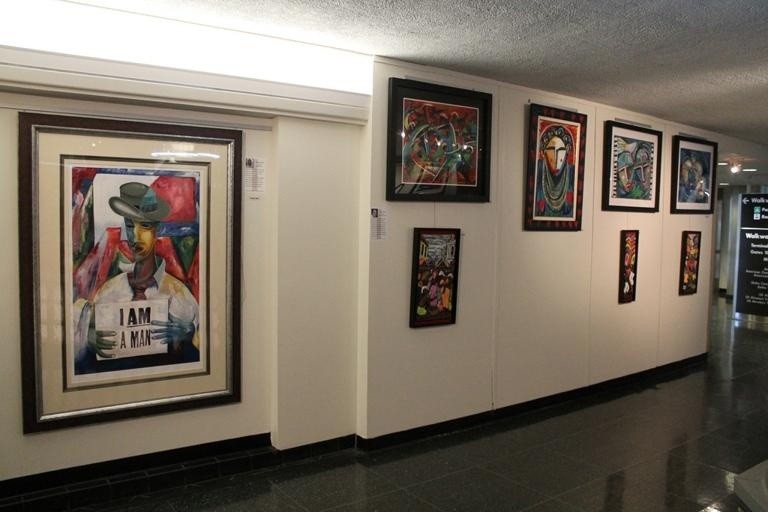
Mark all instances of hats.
[108,182,169,223]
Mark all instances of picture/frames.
[671,131,720,217]
[407,227,460,328]
[618,228,639,305]
[677,228,702,297]
[602,120,662,217]
[522,103,586,234]
[387,77,491,208]
[15,112,243,438]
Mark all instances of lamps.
[726,153,742,176]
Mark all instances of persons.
[78,183,204,372]
[536,124,576,216]
